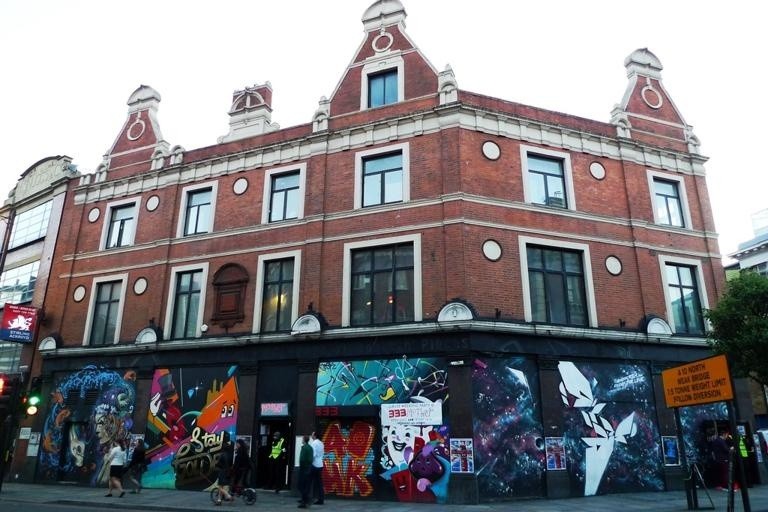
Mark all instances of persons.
[127,439,146,493]
[103,438,128,497]
[706,428,753,492]
[213,438,252,507]
[267,430,324,508]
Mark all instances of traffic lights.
[0,375,14,413]
[26,376,43,418]
[16,390,29,425]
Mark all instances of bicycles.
[208,476,259,506]
